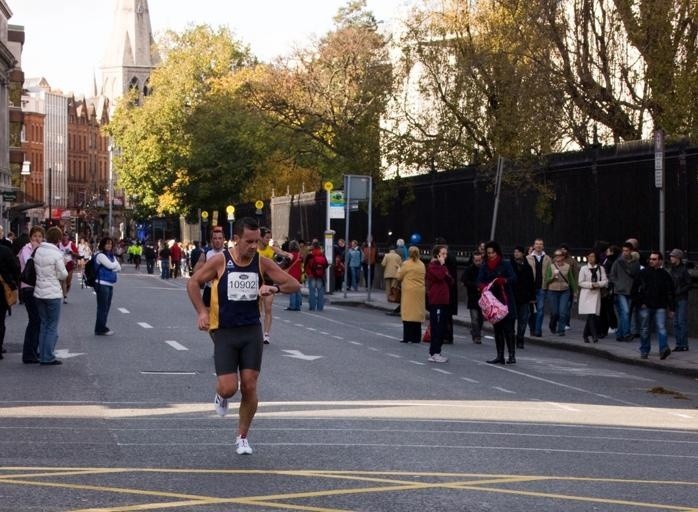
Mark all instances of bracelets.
[275,284,280,294]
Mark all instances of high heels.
[591,333,601,345]
[582,334,590,344]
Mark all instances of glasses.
[648,257,658,262]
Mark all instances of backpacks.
[476,274,512,325]
[307,257,327,278]
[82,252,101,290]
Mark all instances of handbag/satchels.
[0,273,18,307]
[18,248,38,289]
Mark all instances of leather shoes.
[505,358,518,365]
[485,356,505,364]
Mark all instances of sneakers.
[214,391,229,418]
[659,345,671,361]
[640,351,650,361]
[673,345,690,352]
[233,434,253,456]
[62,295,69,304]
[94,329,115,336]
[427,352,449,366]
[474,335,483,345]
[38,360,63,365]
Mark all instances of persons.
[461,252,484,345]
[527,238,547,340]
[434,237,457,317]
[95,239,121,338]
[558,247,577,312]
[624,240,642,261]
[638,252,674,361]
[283,242,304,312]
[304,243,328,311]
[604,247,615,272]
[504,245,535,347]
[78,235,85,268]
[0,226,19,357]
[670,250,693,356]
[83,242,91,264]
[364,238,376,290]
[196,231,230,308]
[335,239,349,286]
[347,240,362,293]
[472,243,486,254]
[395,247,425,346]
[546,250,573,334]
[573,253,607,342]
[481,243,519,365]
[112,236,200,277]
[426,245,453,364]
[256,227,293,345]
[188,217,301,455]
[40,228,69,366]
[613,244,637,341]
[21,226,46,364]
[394,238,410,264]
[382,248,399,302]
[61,233,76,303]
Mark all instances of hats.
[668,249,684,258]
